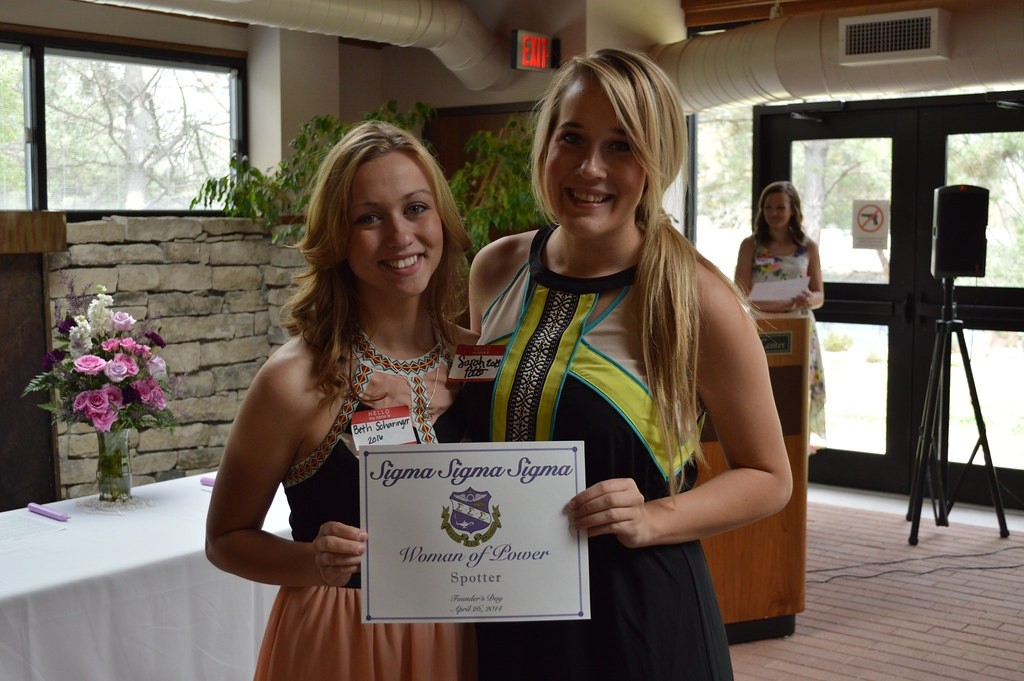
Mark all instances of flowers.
[18,268,180,437]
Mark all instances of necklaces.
[359,315,441,420]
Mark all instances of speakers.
[930,185,989,280]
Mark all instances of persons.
[469,49,792,681]
[736,180,827,458]
[205,122,483,681]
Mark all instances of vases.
[95,429,132,502]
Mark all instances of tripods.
[906,277,1010,545]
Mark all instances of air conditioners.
[837,7,951,68]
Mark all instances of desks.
[0,468,291,681]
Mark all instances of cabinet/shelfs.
[694,313,813,644]
[0,207,66,514]
